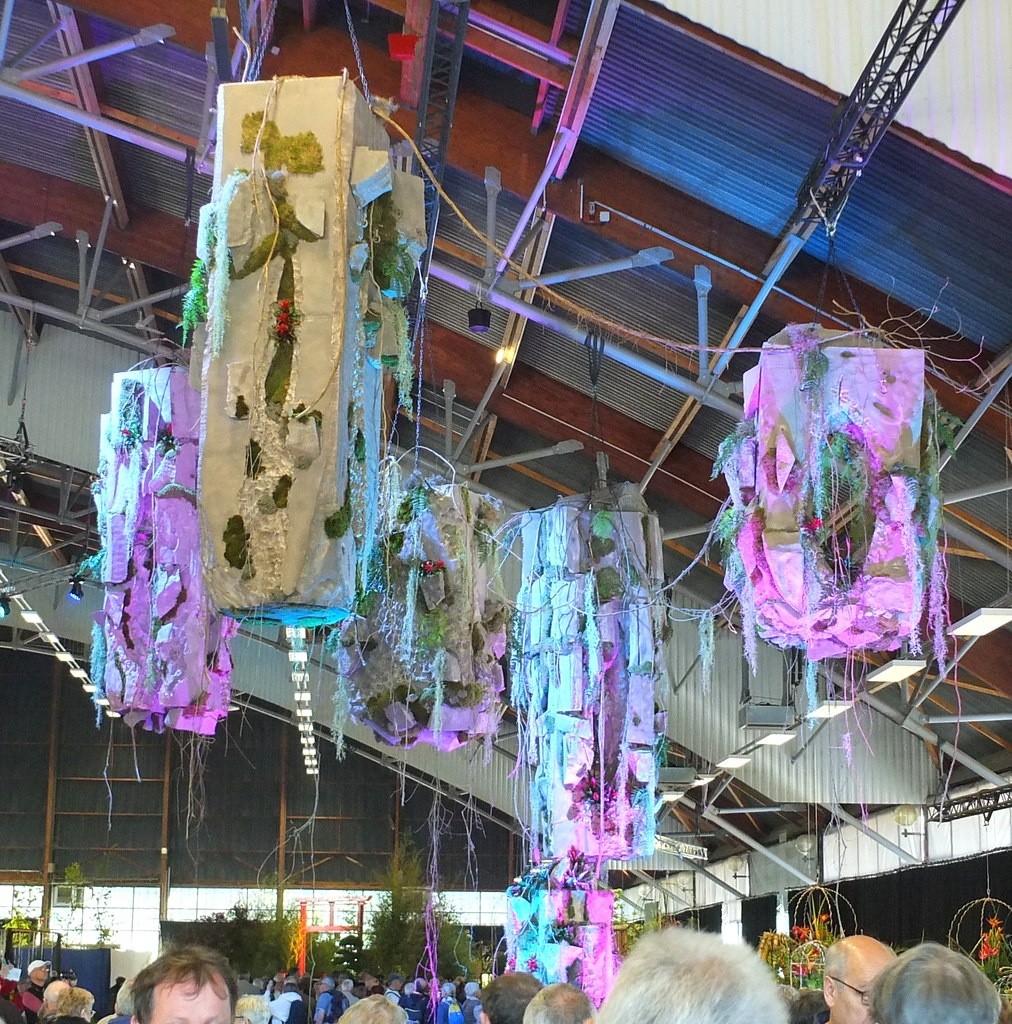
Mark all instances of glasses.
[35,967,49,972]
[831,976,869,1006]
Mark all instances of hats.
[28,960,50,976]
[388,973,401,982]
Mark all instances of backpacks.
[320,989,350,1024]
[282,998,305,1024]
[443,1001,465,1024]
[470,999,484,1024]
[404,993,429,1024]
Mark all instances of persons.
[0,959,96,1024]
[96,927,1012,1024]
